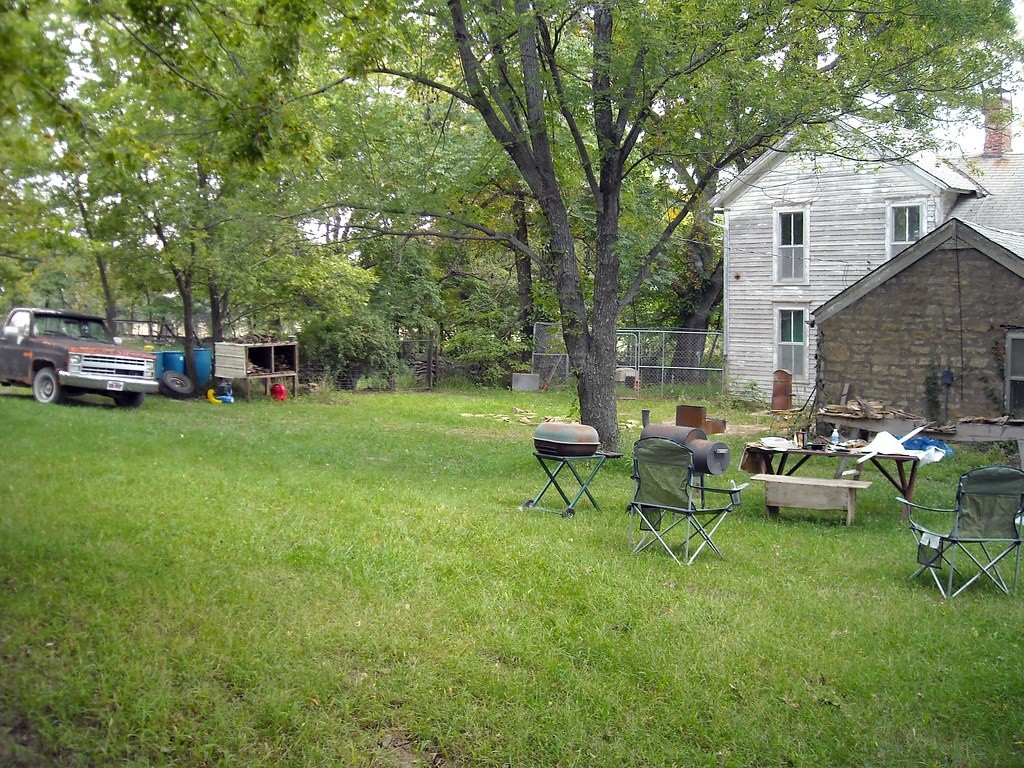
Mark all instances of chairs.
[624,436,733,567]
[894,465,1024,600]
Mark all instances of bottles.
[832,429,840,443]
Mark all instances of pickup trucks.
[0,307,160,408]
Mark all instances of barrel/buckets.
[190,348,211,392]
[163,351,184,374]
[146,352,164,379]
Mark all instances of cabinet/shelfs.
[214,341,298,403]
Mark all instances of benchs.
[751,473,872,527]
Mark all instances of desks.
[738,440,953,518]
[525,453,623,520]
